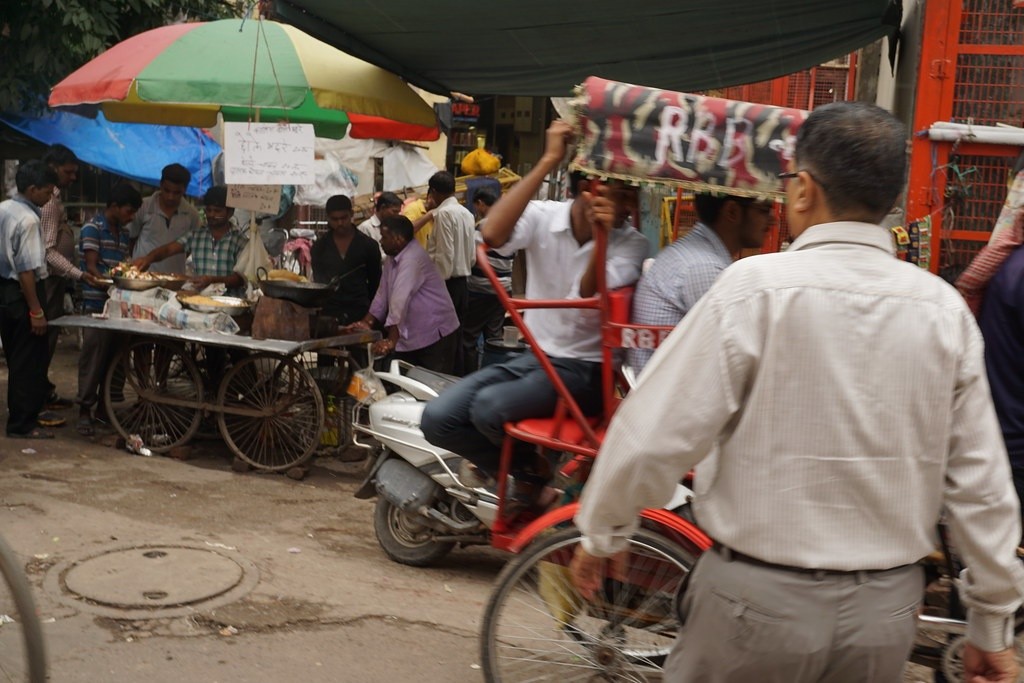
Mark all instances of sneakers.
[33,411,67,426]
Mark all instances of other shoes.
[186,391,209,400]
[519,470,584,526]
[501,468,556,526]
[95,413,132,427]
[77,418,97,435]
[43,393,75,409]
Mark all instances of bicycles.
[478,500,968,683]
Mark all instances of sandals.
[7,427,55,439]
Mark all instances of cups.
[503,326,520,346]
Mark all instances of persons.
[569,102,1023,682]
[0,145,1023,510]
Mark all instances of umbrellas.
[45,15,441,141]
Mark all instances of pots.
[109,267,187,291]
[176,294,261,318]
[256,266,341,306]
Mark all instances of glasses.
[778,170,827,191]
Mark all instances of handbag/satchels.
[347,343,387,405]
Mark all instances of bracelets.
[30,309,45,319]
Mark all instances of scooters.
[347,358,501,567]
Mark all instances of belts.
[712,540,912,576]
